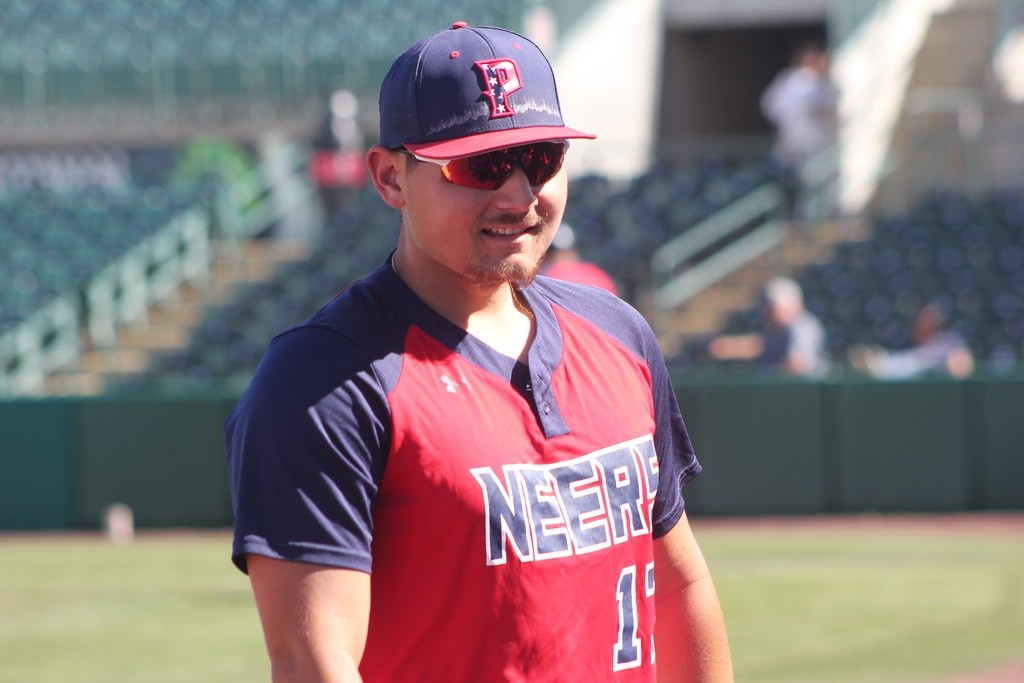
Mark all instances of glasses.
[394,139,570,191]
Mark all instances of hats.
[379,22,597,160]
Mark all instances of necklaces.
[509,283,535,319]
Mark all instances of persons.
[760,41,838,215]
[849,300,974,382]
[227,21,735,683]
[538,224,620,298]
[708,276,832,380]
[310,88,370,225]
[168,133,282,240]
